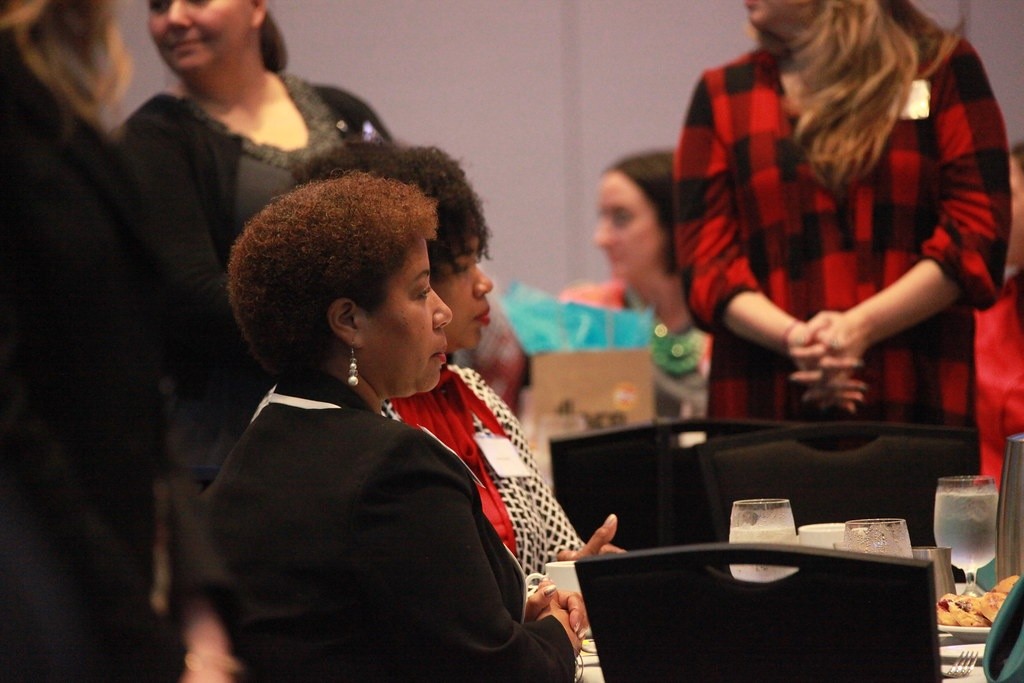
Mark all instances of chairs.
[550,416,803,554]
[694,423,974,547]
[575,543,942,683]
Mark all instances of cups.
[525,561,581,595]
[912,546,958,602]
[798,523,846,551]
[844,519,913,560]
[729,499,799,583]
[997,431,1024,584]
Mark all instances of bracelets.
[185,652,244,672]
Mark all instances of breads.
[937,575,1020,627]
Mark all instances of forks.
[941,651,979,678]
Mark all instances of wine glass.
[933,476,998,598]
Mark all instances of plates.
[937,623,992,642]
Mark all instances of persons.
[0,0,1024,683]
[173,168,592,683]
[670,0,1012,471]
[292,140,626,682]
